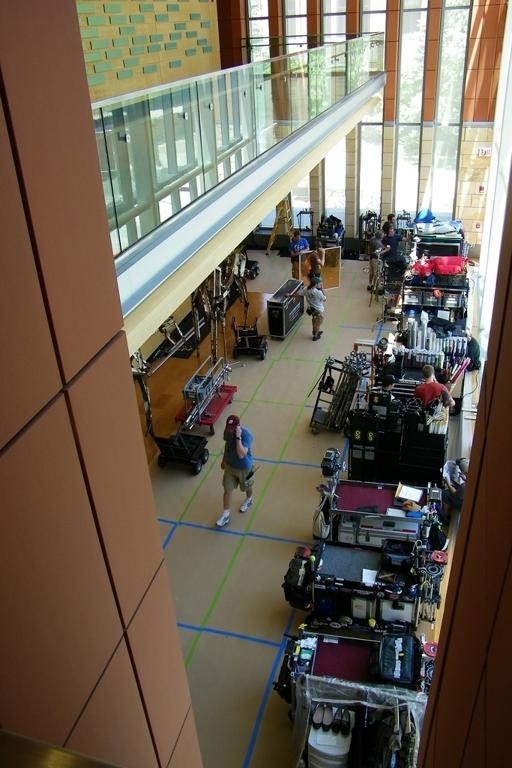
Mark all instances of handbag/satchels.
[313,508,330,539]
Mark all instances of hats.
[383,374,399,383]
[227,415,239,429]
[311,276,322,283]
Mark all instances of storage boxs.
[404,290,461,309]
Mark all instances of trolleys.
[150,433,210,475]
[230,317,268,359]
[309,351,370,434]
[240,246,261,281]
[173,311,237,434]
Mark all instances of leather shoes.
[312,703,350,735]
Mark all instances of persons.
[466,331,480,371]
[443,457,470,522]
[361,374,397,411]
[414,365,456,410]
[289,230,310,279]
[308,241,325,279]
[286,276,327,341]
[367,214,413,291]
[216,414,256,527]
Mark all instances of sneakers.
[313,331,322,341]
[216,514,230,526]
[240,498,253,512]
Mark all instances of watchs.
[235,436,242,440]
